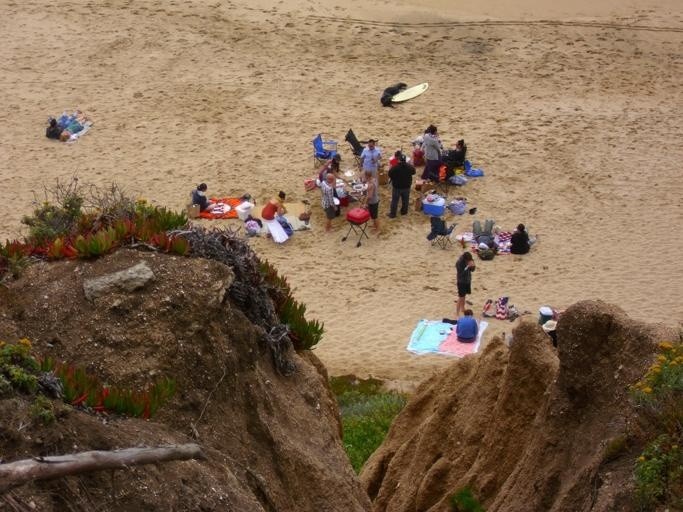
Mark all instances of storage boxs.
[233,201,256,221]
[335,185,351,208]
[420,197,446,216]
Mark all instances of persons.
[321,173,337,231]
[388,124,467,218]
[364,170,380,231]
[262,190,285,220]
[472,219,498,251]
[360,139,382,177]
[454,252,475,316]
[456,309,478,343]
[191,183,213,209]
[319,153,348,199]
[510,223,530,255]
[50,110,86,137]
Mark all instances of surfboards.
[390,82,428,102]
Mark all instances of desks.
[342,220,369,247]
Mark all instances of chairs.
[343,128,378,167]
[311,134,337,167]
[426,159,455,196]
[448,144,466,170]
[427,217,455,249]
